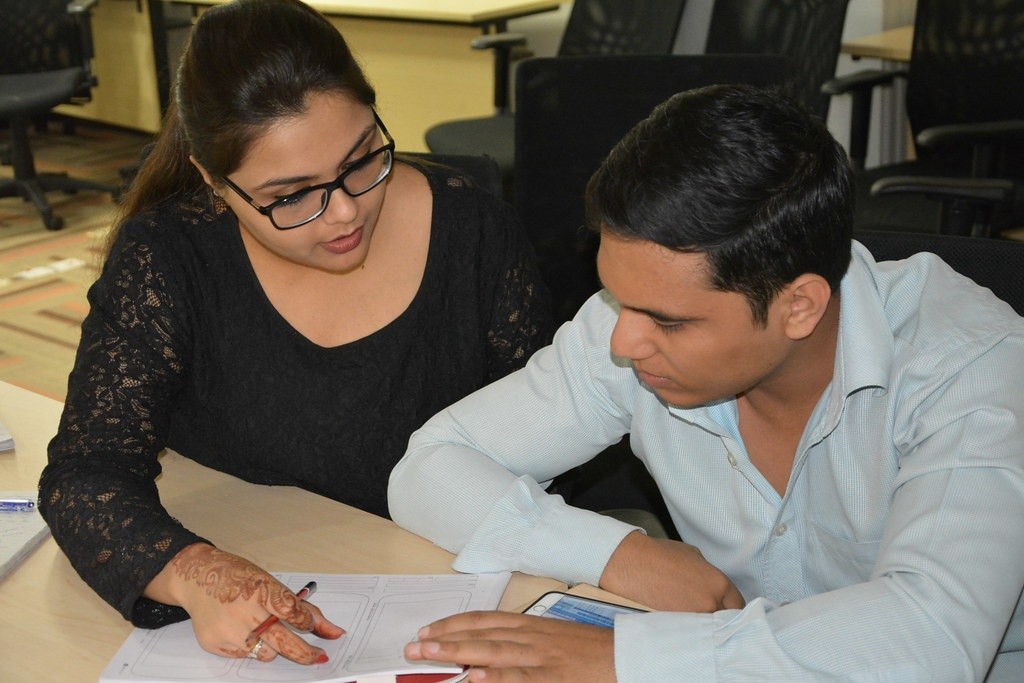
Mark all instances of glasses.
[220,106,395,231]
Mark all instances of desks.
[0,379,656,683]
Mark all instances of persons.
[36,0,659,666]
[382,83,1022,680]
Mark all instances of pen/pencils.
[0,500,34,512]
[245,581,316,642]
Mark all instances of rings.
[247,639,263,659]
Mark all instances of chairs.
[424,0,1024,243]
[0,0,100,230]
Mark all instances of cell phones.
[521,591,650,632]
